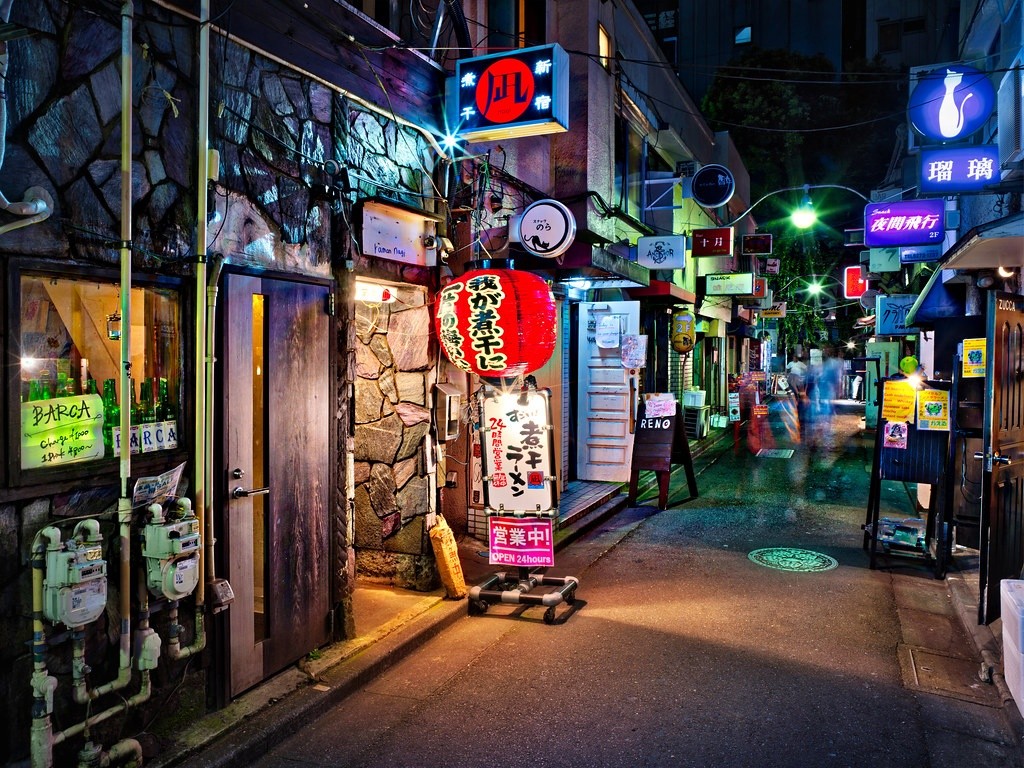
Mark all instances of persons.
[785,356,807,397]
[891,355,919,379]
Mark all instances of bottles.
[156,377,175,422]
[27,380,41,402]
[137,382,156,425]
[88,379,120,453]
[41,381,52,400]
[67,378,75,397]
[145,378,155,406]
[56,373,66,398]
[130,379,140,426]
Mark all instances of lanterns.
[671,307,696,355]
[433,258,556,385]
